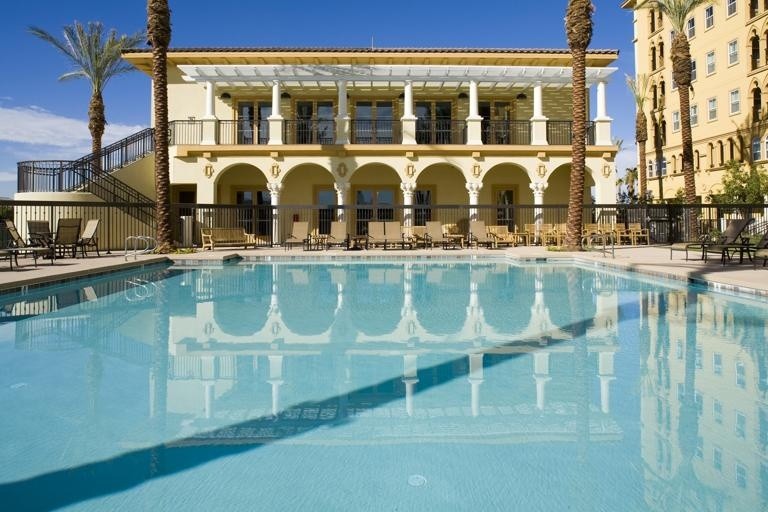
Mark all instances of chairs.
[0,218,100,270]
[284,222,651,252]
[671,218,768,271]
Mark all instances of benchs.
[200,227,256,252]
